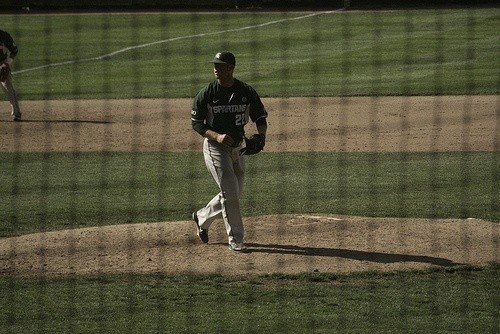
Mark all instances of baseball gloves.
[238,133,265,156]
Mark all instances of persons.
[0,30,21,121]
[192,51,268,252]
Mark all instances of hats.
[207,51,236,65]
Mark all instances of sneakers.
[192,212,208,243]
[229,243,247,252]
[13,113,22,120]
